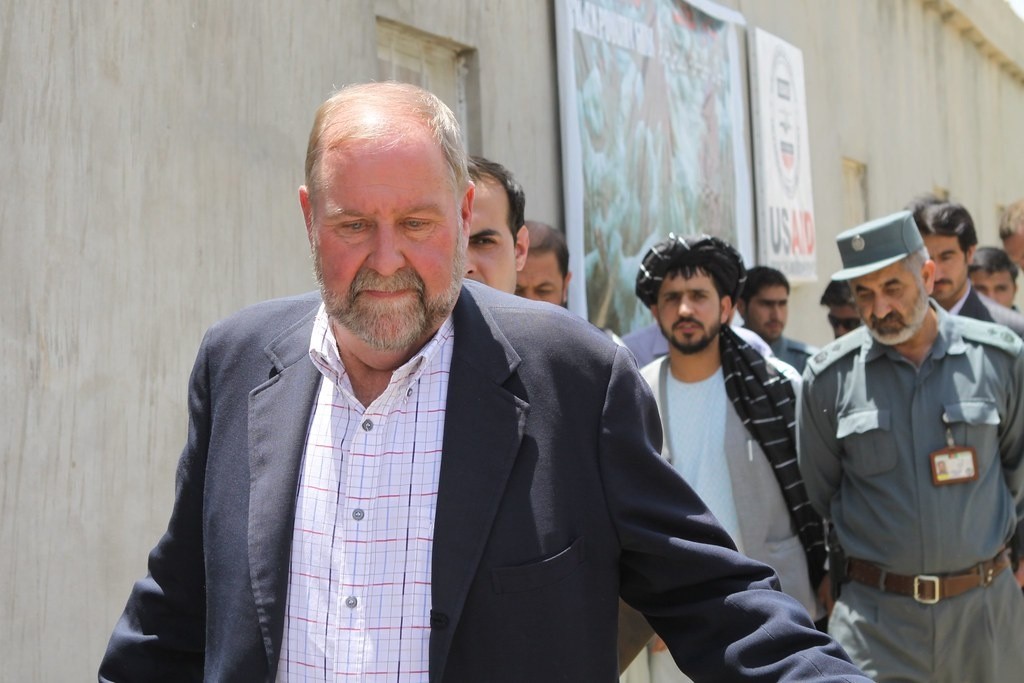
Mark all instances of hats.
[831,210,924,280]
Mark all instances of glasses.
[828,314,867,332]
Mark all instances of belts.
[841,547,1011,605]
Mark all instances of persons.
[936,462,949,474]
[96,80,879,683]
[465,157,1024,683]
